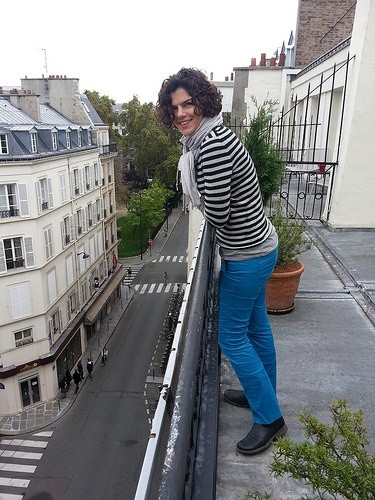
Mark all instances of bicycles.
[159,291,184,341]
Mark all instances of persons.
[59,376,67,399]
[163,272,168,286]
[78,360,85,382]
[156,68,288,455]
[66,370,72,390]
[128,267,132,280]
[87,358,93,381]
[101,346,108,366]
[73,370,81,393]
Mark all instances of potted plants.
[266,197,318,313]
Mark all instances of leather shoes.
[224,389,250,408]
[237,416,288,454]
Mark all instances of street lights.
[138,178,154,261]
